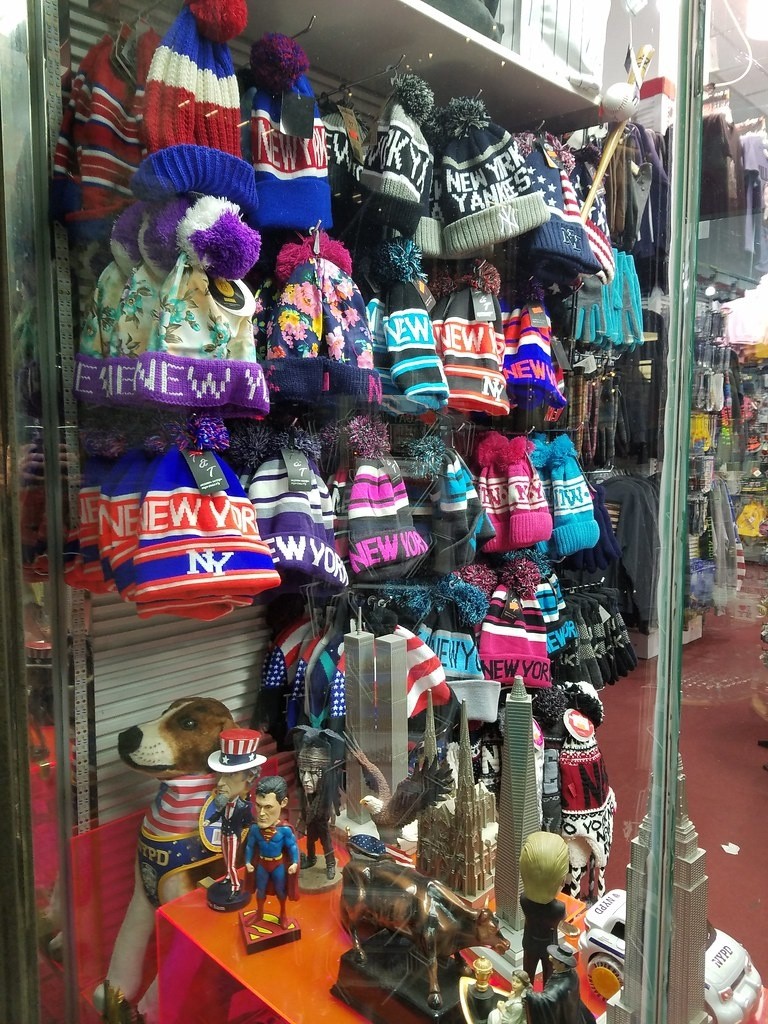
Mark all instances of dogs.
[90,693,259,1023]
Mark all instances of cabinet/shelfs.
[155,835,607,1024]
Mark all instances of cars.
[579,886,763,1024]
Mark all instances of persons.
[488,970,533,1024]
[201,730,299,930]
[294,767,336,880]
[519,832,569,991]
[526,945,596,1024]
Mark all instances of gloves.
[553,244,648,681]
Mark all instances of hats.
[51,0,619,913]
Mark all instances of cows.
[337,858,512,1013]
